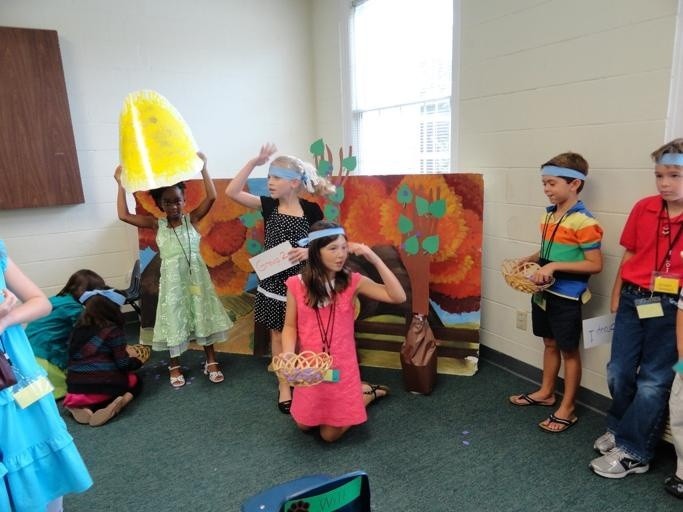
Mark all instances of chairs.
[239,470,374,512]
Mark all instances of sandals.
[203,358,224,383]
[361,380,390,398]
[168,364,187,387]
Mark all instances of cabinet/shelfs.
[1,23,84,210]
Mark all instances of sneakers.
[663,472,683,499]
[593,431,616,454]
[587,445,650,479]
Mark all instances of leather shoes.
[277,384,293,414]
[64,406,92,423]
[88,396,123,426]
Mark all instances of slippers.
[538,411,578,432]
[509,392,555,406]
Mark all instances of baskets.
[501,256,556,294]
[266,350,334,388]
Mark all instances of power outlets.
[513,309,526,331]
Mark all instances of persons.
[275,220,406,444]
[27,269,105,363]
[0,236,95,512]
[113,151,227,386]
[53,288,137,427]
[226,142,324,412]
[508,153,603,434]
[589,133,683,477]
[662,287,683,495]
